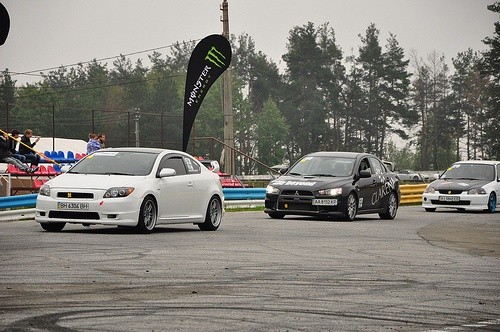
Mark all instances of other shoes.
[30,167,39,173]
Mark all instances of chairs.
[6,151,86,190]
[220,178,242,187]
[334,163,345,175]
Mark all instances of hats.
[11,130,20,134]
[25,129,32,133]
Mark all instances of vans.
[199,159,220,173]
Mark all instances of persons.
[8,131,26,163]
[87,134,101,155]
[0,130,39,173]
[341,164,360,178]
[19,129,41,170]
[97,133,105,149]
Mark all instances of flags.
[183,35,232,152]
[0,2,10,45]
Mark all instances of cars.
[383,161,444,184]
[263,151,401,222]
[34,146,226,235]
[266,165,289,176]
[421,160,500,214]
